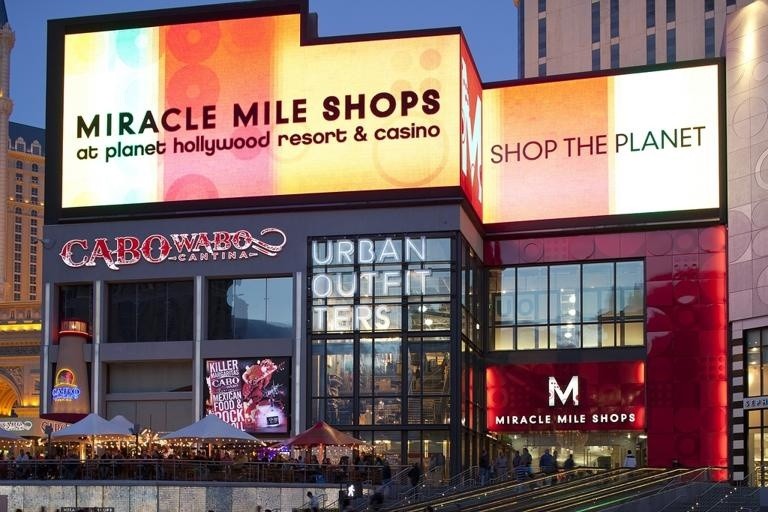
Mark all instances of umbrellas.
[108,415,145,445]
[277,420,366,464]
[164,414,267,447]
[44,413,131,458]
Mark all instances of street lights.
[41,421,52,457]
[129,422,140,458]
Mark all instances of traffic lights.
[347,480,363,500]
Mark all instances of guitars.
[241,360,286,397]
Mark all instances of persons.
[623,449,637,480]
[1,444,393,482]
[306,491,320,512]
[428,451,446,480]
[367,485,384,512]
[672,458,681,469]
[478,445,558,493]
[338,487,351,512]
[563,453,577,481]
[407,460,421,500]
